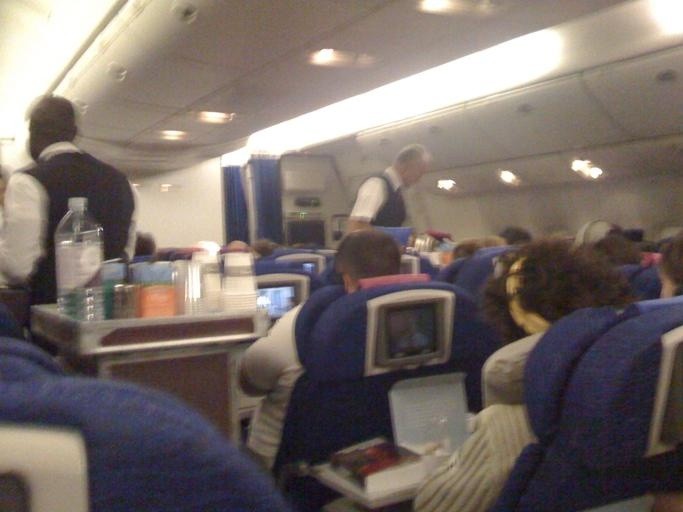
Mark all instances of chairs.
[411,254,439,276]
[238,247,329,322]
[0,337,62,377]
[433,243,519,298]
[273,274,487,506]
[491,297,682,508]
[0,378,295,512]
[617,263,663,300]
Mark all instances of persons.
[344,142,454,243]
[0,95,134,378]
[220,226,683,511]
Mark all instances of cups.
[171,252,257,318]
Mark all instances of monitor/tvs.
[300,261,319,275]
[398,262,414,273]
[379,302,444,367]
[257,279,301,321]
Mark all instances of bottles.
[54,197,171,321]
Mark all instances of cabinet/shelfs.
[31,303,267,451]
